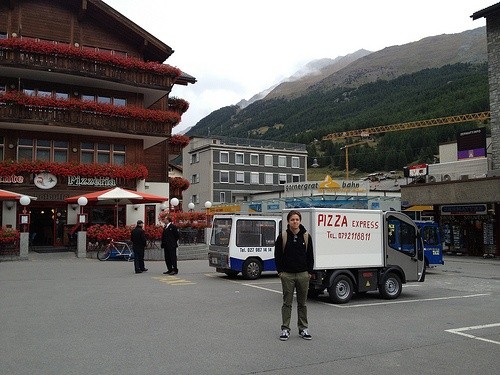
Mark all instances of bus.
[389,217,444,267]
[207,214,282,280]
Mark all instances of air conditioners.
[459,172,473,180]
[426,172,456,183]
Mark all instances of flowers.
[0,158,148,181]
[87,223,163,241]
[0,227,20,242]
[168,134,190,146]
[0,89,189,126]
[159,211,210,229]
[172,177,190,191]
[0,37,183,77]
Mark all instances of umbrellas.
[0,189,37,200]
[65,186,168,228]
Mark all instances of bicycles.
[97,238,136,263]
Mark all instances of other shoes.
[141,267,148,271]
[169,268,178,275]
[163,267,173,274]
[135,269,143,272]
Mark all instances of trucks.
[281,208,425,304]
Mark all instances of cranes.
[322,111,490,141]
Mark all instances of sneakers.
[299,329,312,340]
[280,329,291,340]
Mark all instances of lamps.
[5,200,15,210]
[134,204,139,211]
[71,204,78,211]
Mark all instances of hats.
[137,220,143,224]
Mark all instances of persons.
[131,220,148,273]
[161,217,179,274]
[274,210,315,340]
